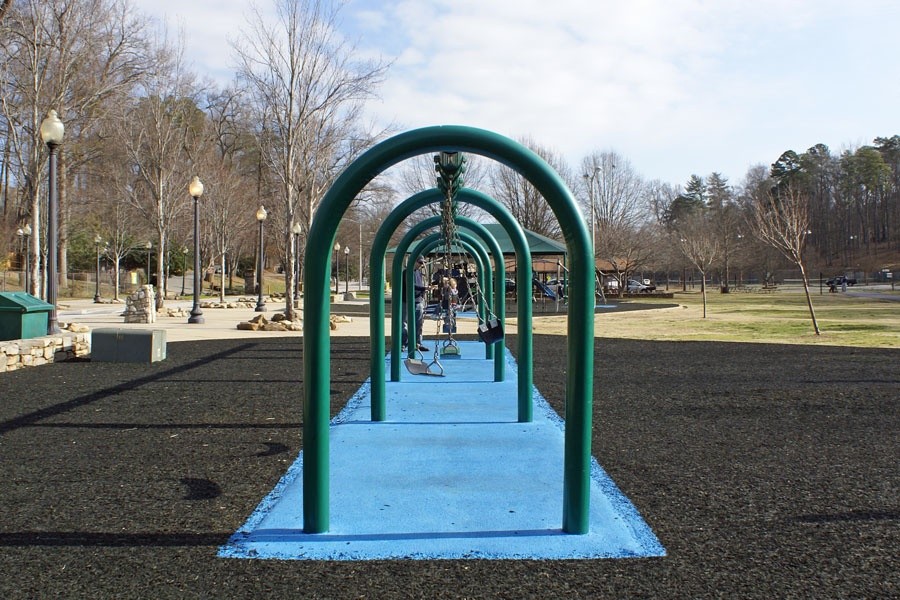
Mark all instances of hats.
[417,255,427,262]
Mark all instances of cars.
[547,278,569,292]
[492,278,516,293]
[624,279,650,294]
[826,275,858,287]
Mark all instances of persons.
[442,278,458,309]
[829,274,847,293]
[402,253,431,352]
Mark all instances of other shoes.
[419,347,429,352]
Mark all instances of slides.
[534,278,562,300]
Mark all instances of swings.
[403,179,503,377]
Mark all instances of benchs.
[758,286,777,293]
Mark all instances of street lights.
[680,238,687,291]
[582,166,603,256]
[40,108,65,335]
[188,175,206,324]
[17,229,23,270]
[293,222,303,300]
[180,246,188,296]
[802,230,812,286]
[145,241,152,284]
[344,246,350,294]
[94,234,102,304]
[342,213,382,292]
[22,223,32,293]
[738,235,746,284]
[334,242,341,294]
[850,235,858,278]
[255,205,268,313]
